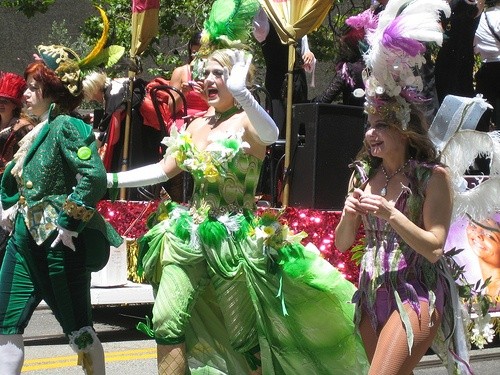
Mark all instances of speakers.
[288,102,368,212]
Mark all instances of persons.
[334,1,455,375]
[76,1,372,375]
[0,4,125,374]
[0,71,36,270]
[467,218,500,306]
[167,1,500,160]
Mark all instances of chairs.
[246,88,286,209]
[148,86,187,199]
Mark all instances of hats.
[0,70,28,108]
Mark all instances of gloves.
[72,162,170,191]
[50,223,79,252]
[223,49,279,145]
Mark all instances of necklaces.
[379,158,412,196]
[215,106,236,120]
